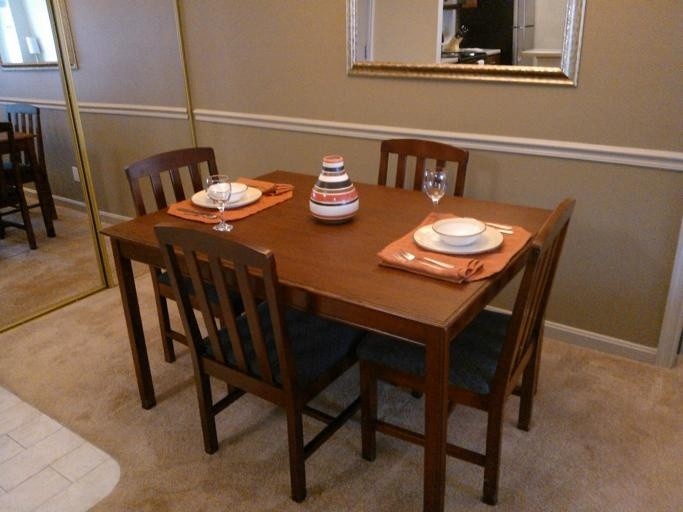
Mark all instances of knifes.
[175,206,217,220]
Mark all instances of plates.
[190,183,263,210]
[412,220,505,256]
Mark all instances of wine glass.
[204,174,235,234]
[421,168,445,223]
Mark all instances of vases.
[306,154,361,226]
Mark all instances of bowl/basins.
[431,216,487,247]
[207,182,247,204]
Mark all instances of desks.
[522,50,562,67]
[0,129,56,240]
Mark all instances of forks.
[397,248,457,269]
[392,253,447,274]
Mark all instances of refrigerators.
[513,0,567,66]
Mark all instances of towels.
[374,240,486,286]
[231,173,296,200]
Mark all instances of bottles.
[441,22,471,53]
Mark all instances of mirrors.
[341,0,585,90]
[48,0,204,289]
[0,0,107,337]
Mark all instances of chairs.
[356,196,579,506]
[0,121,39,251]
[0,104,59,223]
[374,136,472,199]
[122,142,267,403]
[151,221,379,503]
